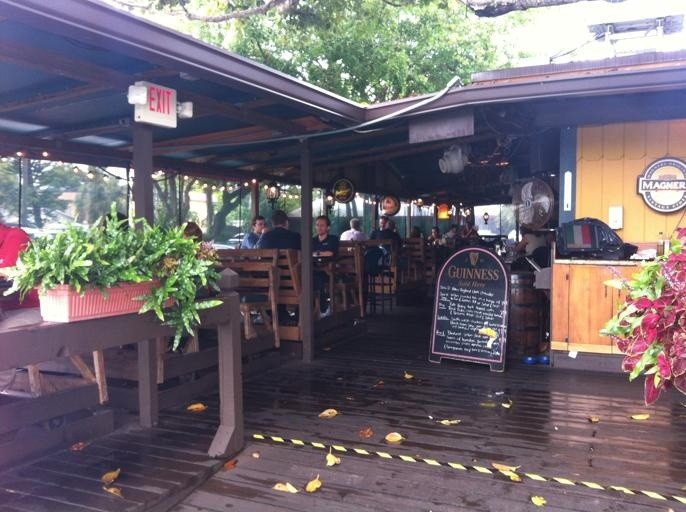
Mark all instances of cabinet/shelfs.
[665,231,671,252]
[655,231,665,257]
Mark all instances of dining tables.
[0,197,227,353]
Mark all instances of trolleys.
[507,176,556,231]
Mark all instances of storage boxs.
[206,221,463,355]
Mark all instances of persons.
[312,216,340,318]
[369,216,388,240]
[445,224,458,238]
[185,221,202,243]
[411,226,421,238]
[376,220,403,251]
[240,216,268,261]
[104,213,129,230]
[256,212,302,322]
[340,218,370,250]
[460,222,478,238]
[0,223,41,310]
[428,227,448,246]
[511,225,554,271]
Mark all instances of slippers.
[362,246,385,276]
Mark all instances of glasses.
[434,198,452,219]
[413,191,424,208]
[482,211,490,224]
[263,174,281,210]
[124,80,195,131]
[322,183,337,215]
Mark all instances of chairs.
[320,307,330,319]
